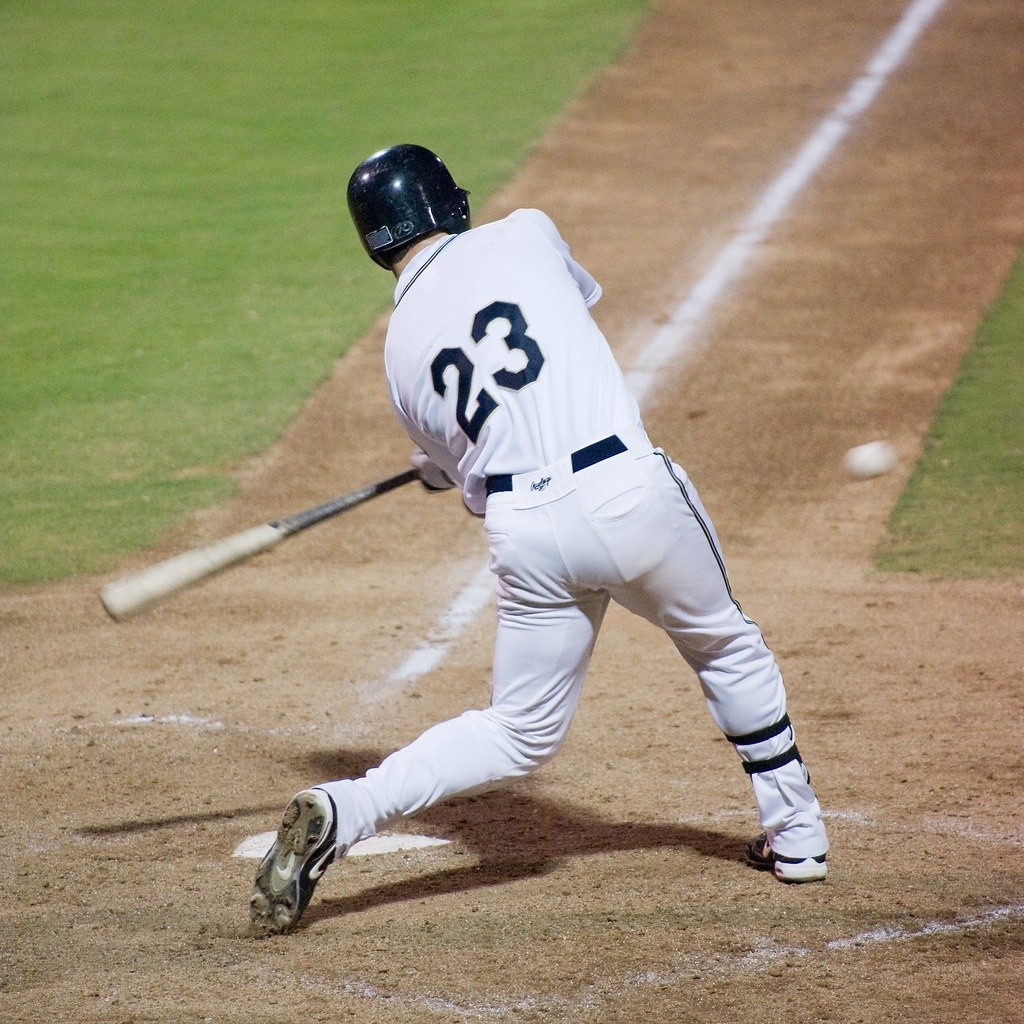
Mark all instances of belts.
[484,436,628,497]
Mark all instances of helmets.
[346,144,470,270]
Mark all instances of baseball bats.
[96,461,420,624]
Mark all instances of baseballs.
[839,440,896,482]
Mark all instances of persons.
[248,146,832,937]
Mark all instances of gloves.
[409,447,458,493]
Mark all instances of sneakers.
[247,788,338,937]
[743,831,828,882]
